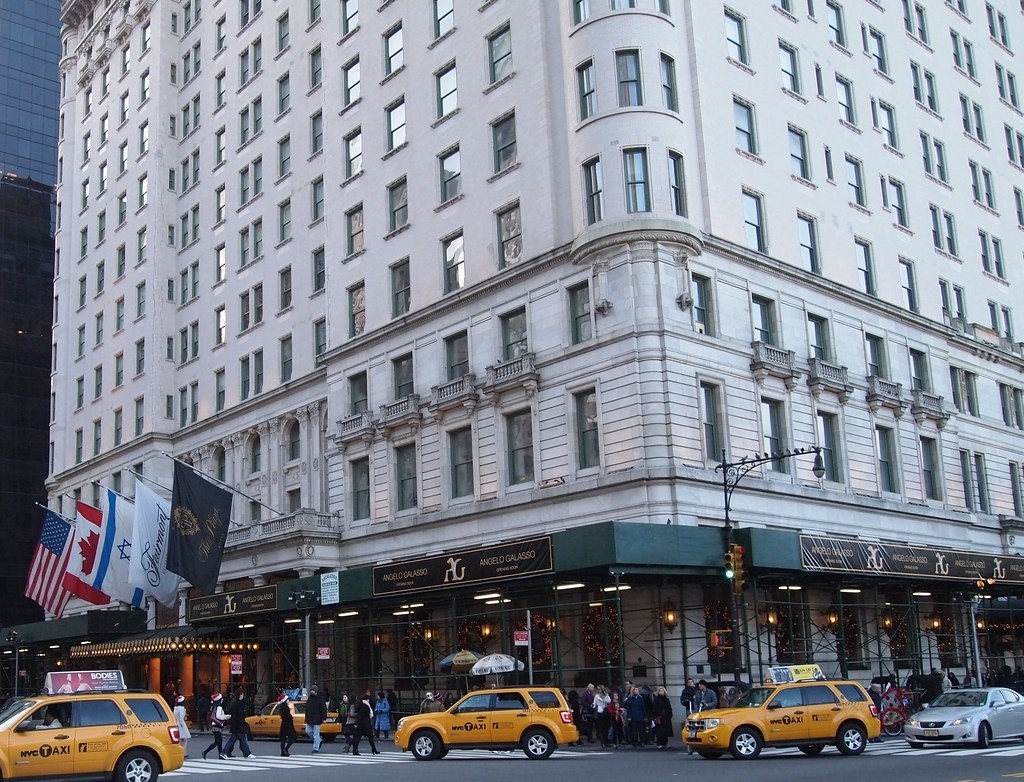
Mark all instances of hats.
[175,696,185,706]
[210,693,222,703]
[310,685,319,693]
[277,695,288,705]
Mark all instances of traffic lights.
[725,553,735,579]
[710,632,725,648]
[734,545,748,593]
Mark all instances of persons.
[547,677,745,755]
[158,675,518,755]
[31,708,64,731]
[865,664,1024,742]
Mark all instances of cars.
[903,687,1024,749]
[245,701,342,742]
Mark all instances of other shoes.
[228,754,236,757]
[319,738,324,750]
[220,753,229,759]
[247,754,256,759]
[201,751,206,760]
[281,753,290,756]
[283,748,288,756]
[312,750,318,753]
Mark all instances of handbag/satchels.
[346,704,357,724]
[653,716,662,724]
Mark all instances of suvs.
[394,685,580,761]
[0,689,184,782]
[682,680,881,760]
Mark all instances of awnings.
[69,619,263,660]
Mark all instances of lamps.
[821,603,839,635]
[481,615,492,643]
[663,597,677,635]
[424,622,435,646]
[546,612,555,634]
[373,627,383,646]
[924,606,942,635]
[878,605,894,639]
[766,601,778,635]
[976,614,985,632]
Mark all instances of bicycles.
[881,692,924,736]
[687,700,703,755]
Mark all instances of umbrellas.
[439,648,486,694]
[469,652,525,688]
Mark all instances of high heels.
[371,748,380,755]
[353,751,360,755]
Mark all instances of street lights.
[715,446,825,683]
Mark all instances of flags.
[23,459,234,622]
